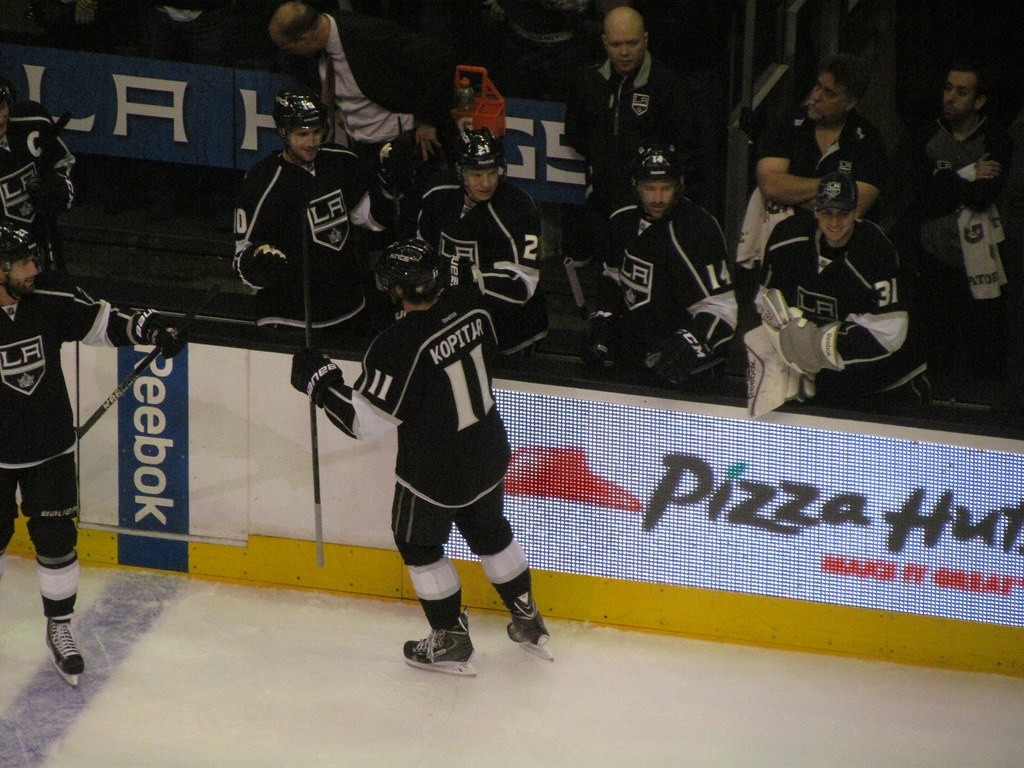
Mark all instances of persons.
[0,0,1023,437]
[289,239,553,679]
[0,222,185,688]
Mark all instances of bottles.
[456,77,475,112]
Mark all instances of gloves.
[132,308,189,359]
[646,326,713,388]
[241,244,286,288]
[753,285,845,372]
[290,346,345,409]
[439,257,477,300]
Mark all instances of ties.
[321,52,336,142]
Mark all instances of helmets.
[273,87,329,129]
[449,126,507,169]
[630,143,684,181]
[0,75,15,115]
[380,237,440,305]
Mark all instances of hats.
[816,172,858,212]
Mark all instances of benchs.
[57,215,1007,414]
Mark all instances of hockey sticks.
[302,199,325,568]
[76,284,220,440]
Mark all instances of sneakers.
[403,610,478,677]
[507,591,555,662]
[45,612,85,686]
